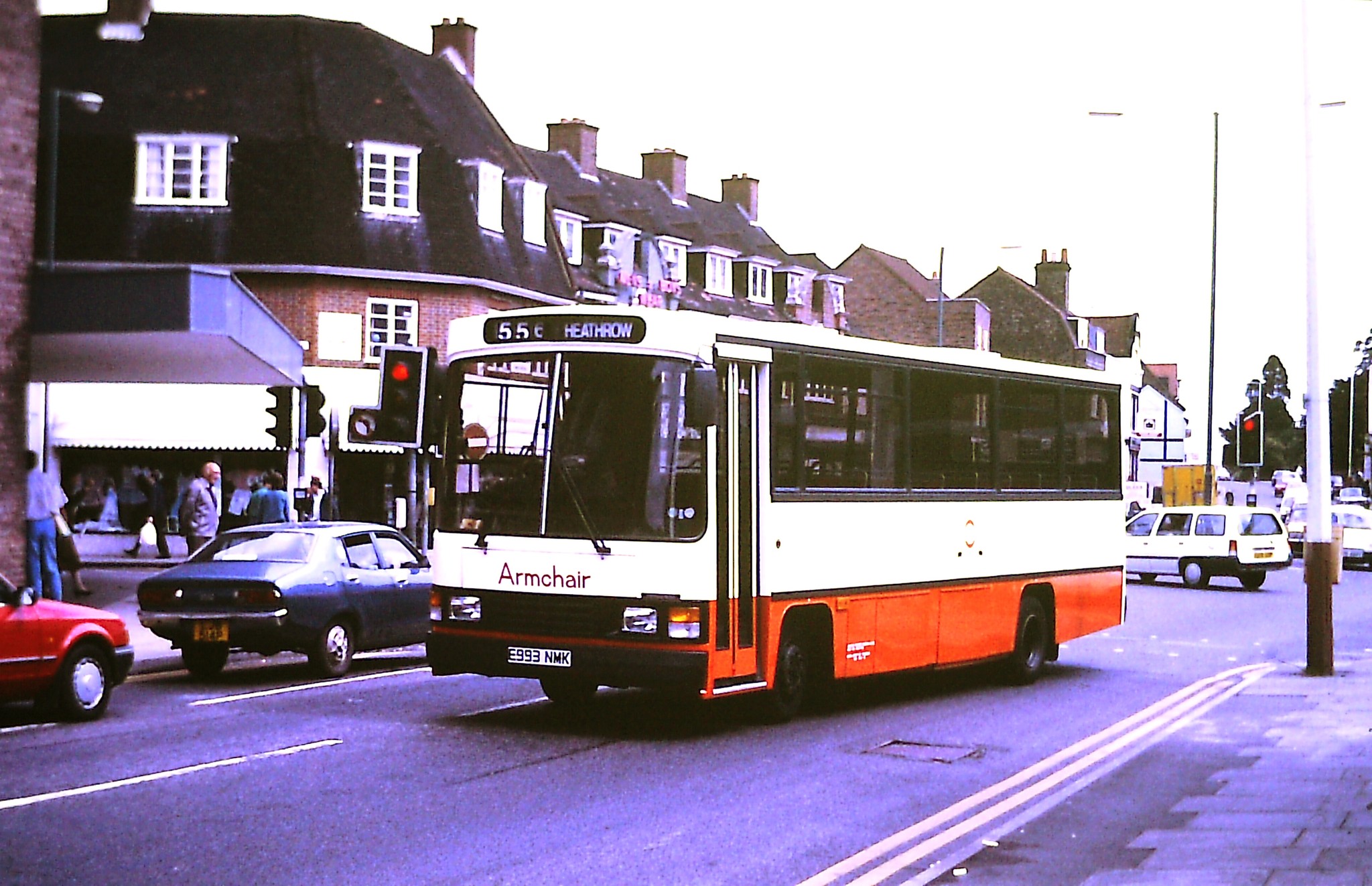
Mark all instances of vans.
[1123,504,1296,590]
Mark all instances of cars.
[136,520,432,677]
[1273,469,1301,497]
[0,573,130,724]
[1330,474,1371,510]
[1288,505,1372,570]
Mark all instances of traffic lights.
[350,346,428,448]
[1236,410,1263,467]
[307,384,327,438]
[263,385,293,449]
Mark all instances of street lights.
[1087,99,1350,505]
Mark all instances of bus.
[427,303,1130,722]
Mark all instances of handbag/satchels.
[139,520,158,546]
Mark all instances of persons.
[62,463,196,533]
[248,471,339,540]
[179,462,221,556]
[24,450,94,601]
[124,471,171,558]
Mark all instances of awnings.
[27,379,289,451]
[461,373,548,455]
[338,400,404,454]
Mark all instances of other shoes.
[124,546,139,559]
